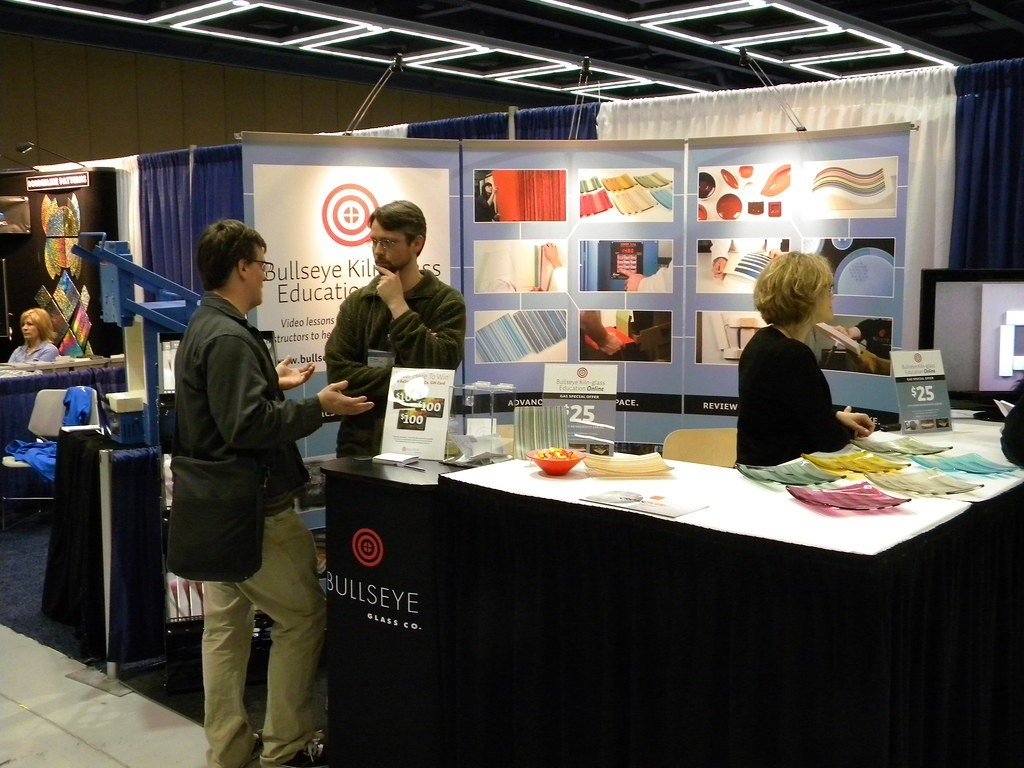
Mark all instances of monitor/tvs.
[920,268,1024,422]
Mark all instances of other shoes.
[244,728,329,768]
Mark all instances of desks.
[438,410,1023,768]
[314,457,471,768]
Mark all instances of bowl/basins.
[526,446,586,476]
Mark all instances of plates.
[580,173,673,216]
[700,172,716,198]
[716,193,742,220]
[583,451,675,474]
[698,203,708,220]
[735,433,1019,512]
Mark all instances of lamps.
[15,142,86,168]
[343,53,404,136]
[567,56,592,140]
[738,46,807,131]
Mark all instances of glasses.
[367,239,414,249]
[236,256,272,272]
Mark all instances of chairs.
[662,428,738,468]
[0,388,99,533]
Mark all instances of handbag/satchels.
[166,455,267,583]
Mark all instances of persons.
[711,239,784,283]
[735,250,875,467]
[835,319,892,360]
[170,220,374,768]
[579,310,623,361]
[619,258,673,292]
[326,200,467,461]
[541,243,566,292]
[8,308,58,362]
[475,182,500,221]
